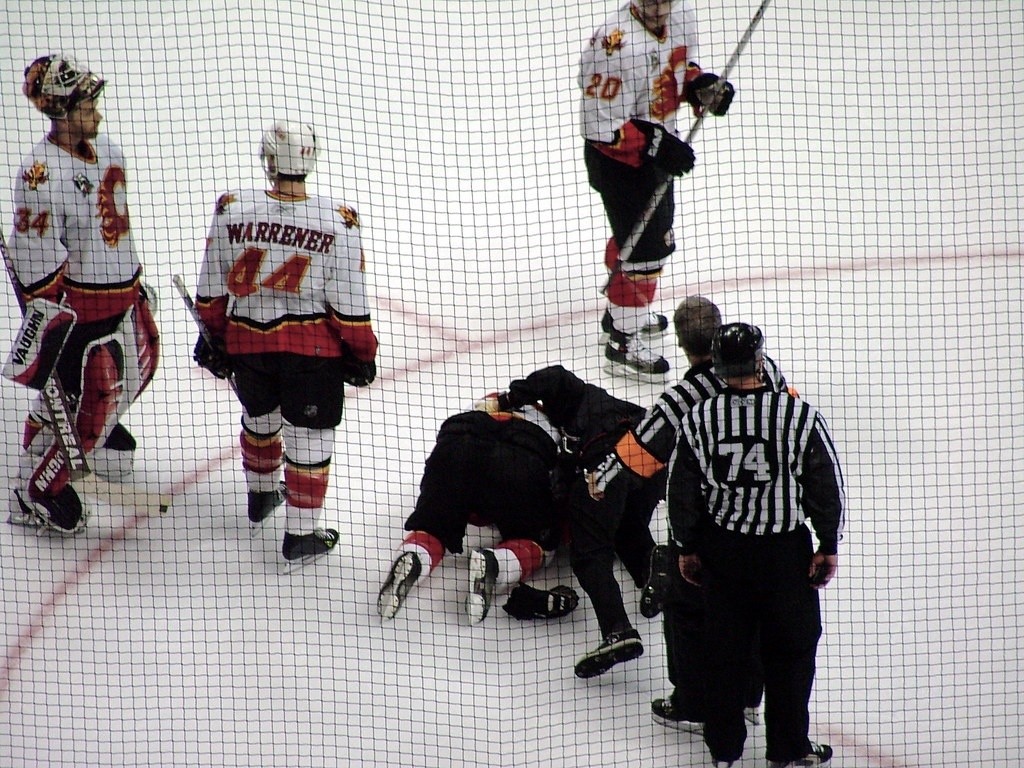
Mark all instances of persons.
[577,0,735,384]
[2,53,163,535]
[379,296,850,767]
[193,122,377,574]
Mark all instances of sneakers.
[710,755,734,768]
[598,300,670,345]
[247,480,286,538]
[743,681,764,725]
[766,740,833,768]
[575,629,645,678]
[282,528,339,575]
[639,544,668,617]
[650,698,704,731]
[602,320,669,383]
[465,548,501,627]
[377,543,431,626]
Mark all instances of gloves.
[475,391,510,421]
[194,326,234,379]
[504,582,582,623]
[345,353,376,388]
[683,73,735,117]
[635,118,696,175]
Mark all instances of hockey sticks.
[173,276,240,403]
[600,2,769,297]
[0,231,90,482]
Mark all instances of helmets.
[23,57,106,121]
[259,120,322,181]
[710,324,765,390]
